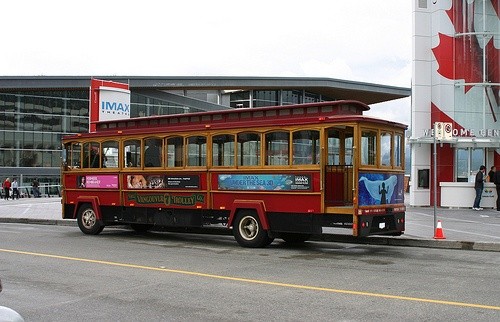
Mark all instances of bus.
[59,99,409,248]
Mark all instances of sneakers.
[472,207,483,211]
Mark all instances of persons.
[79,176,86,188]
[144,138,162,167]
[32,178,41,198]
[3,177,11,200]
[494,171,500,211]
[84,149,104,168]
[488,166,496,182]
[472,165,488,211]
[11,178,20,199]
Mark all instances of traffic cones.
[432,219,448,239]
